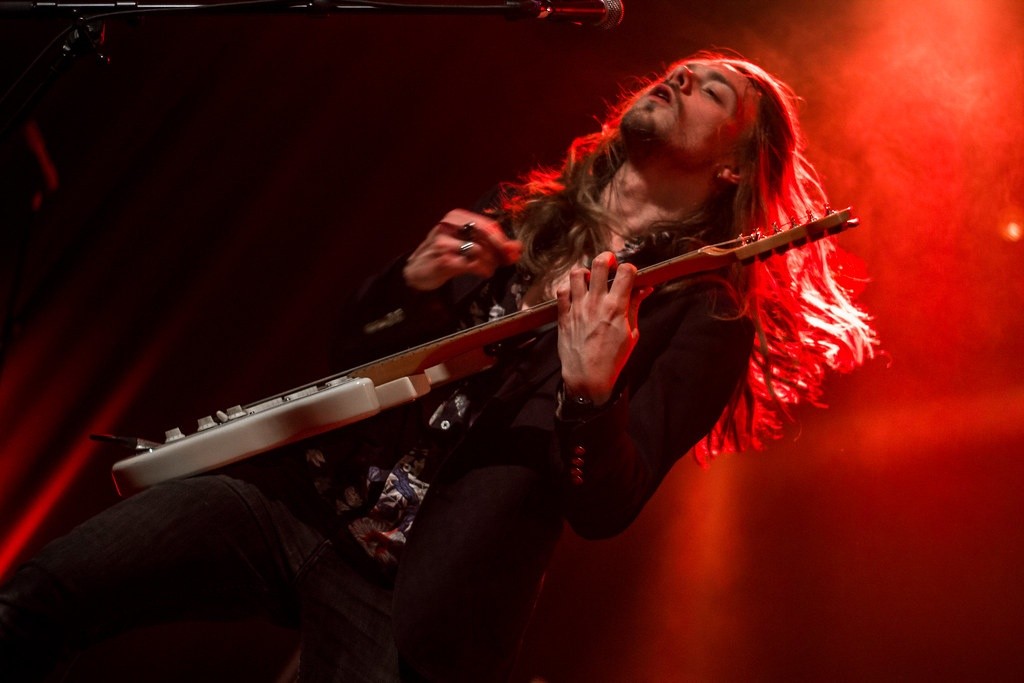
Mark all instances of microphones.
[504,0,623,31]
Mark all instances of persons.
[1,52,870,683]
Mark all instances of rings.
[460,222,476,240]
[460,242,473,256]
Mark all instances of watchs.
[566,395,596,410]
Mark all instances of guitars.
[108,204,862,502]
